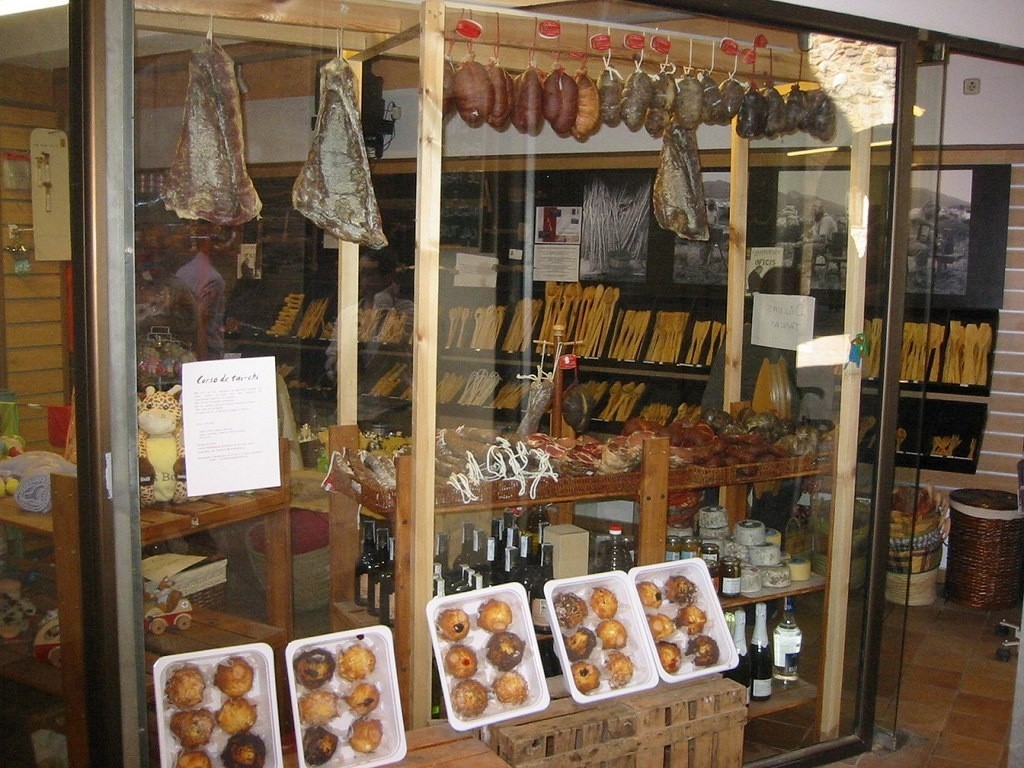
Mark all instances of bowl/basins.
[151,642,284,768]
[627,558,740,684]
[543,569,660,704]
[284,625,408,768]
[425,581,550,732]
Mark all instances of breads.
[437,589,633,717]
[638,578,721,672]
[166,646,382,768]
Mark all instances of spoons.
[945,321,990,385]
[443,281,644,424]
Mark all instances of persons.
[790,199,839,272]
[323,250,413,388]
[173,222,228,359]
[903,200,940,289]
[704,199,719,231]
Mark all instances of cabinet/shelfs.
[0,259,997,767]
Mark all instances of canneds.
[664,536,741,599]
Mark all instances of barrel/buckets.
[244,519,331,612]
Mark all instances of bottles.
[723,610,752,708]
[772,596,802,689]
[353,503,554,635]
[748,602,773,700]
[601,526,633,574]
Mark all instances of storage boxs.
[543,523,589,580]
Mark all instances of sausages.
[332,426,537,490]
